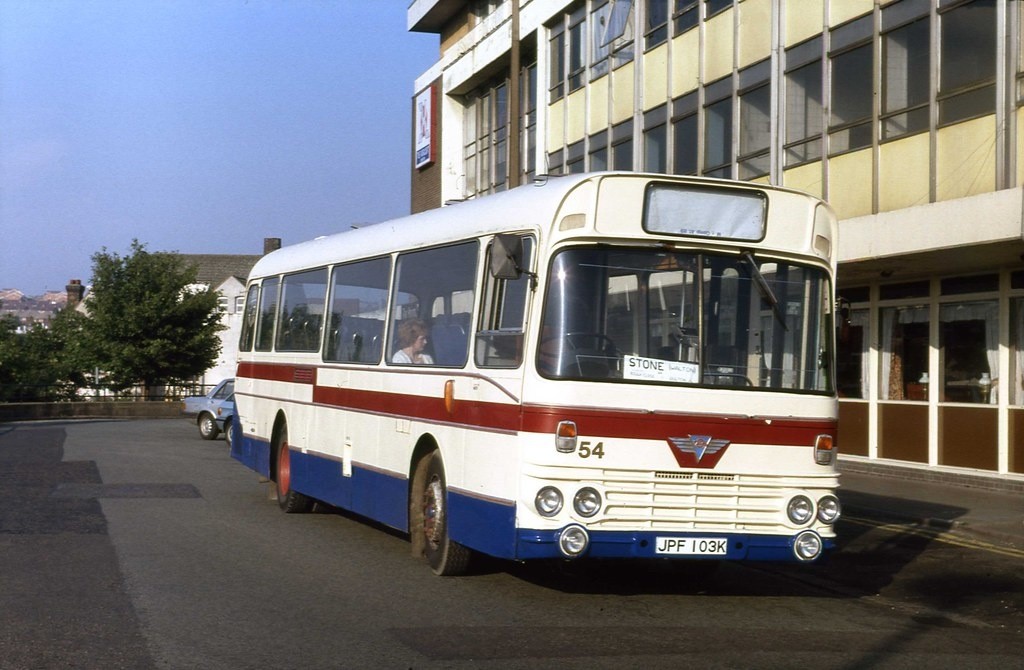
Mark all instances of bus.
[230,169,842,579]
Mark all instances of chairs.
[248,307,621,387]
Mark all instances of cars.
[181,378,234,445]
[213,392,233,448]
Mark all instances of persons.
[392,320,433,364]
